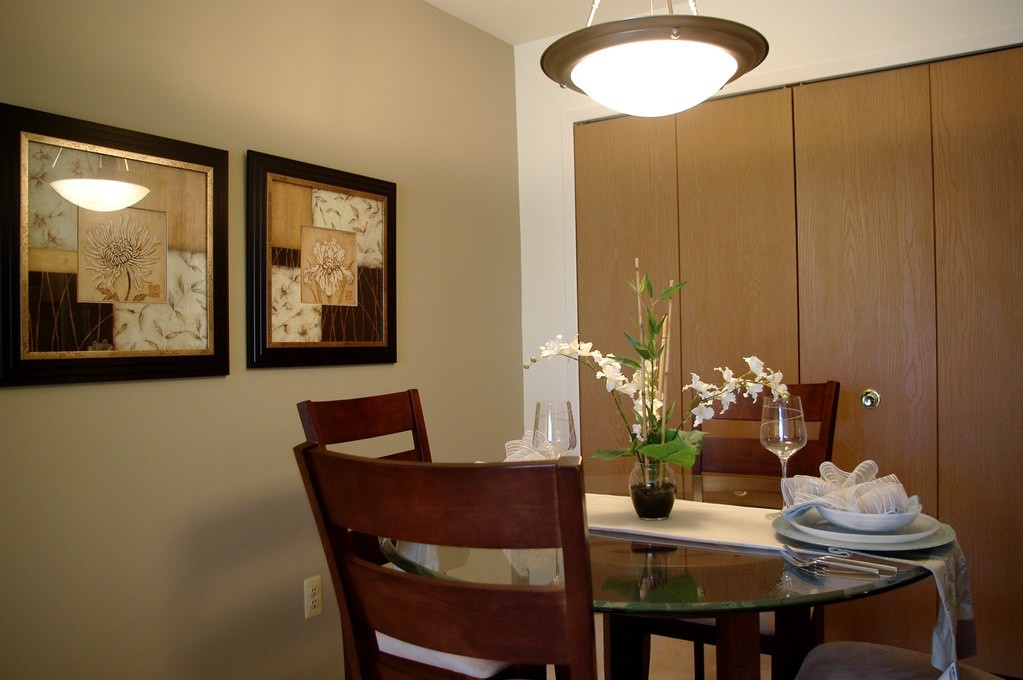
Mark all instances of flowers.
[522,271,792,490]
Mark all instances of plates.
[789,511,941,543]
[771,514,956,552]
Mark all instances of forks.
[783,544,899,573]
[777,549,879,576]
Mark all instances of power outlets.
[303,575,324,621]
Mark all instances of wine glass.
[761,396,807,521]
[533,401,577,461]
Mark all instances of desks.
[378,475,956,680]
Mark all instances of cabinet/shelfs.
[574,43,1023,680]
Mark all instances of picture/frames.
[0,101,229,389]
[245,147,398,370]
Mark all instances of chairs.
[292,388,599,680]
[603,381,841,680]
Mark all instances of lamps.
[45,146,152,211]
[540,1,770,118]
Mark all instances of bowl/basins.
[815,503,923,531]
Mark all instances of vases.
[628,461,678,521]
